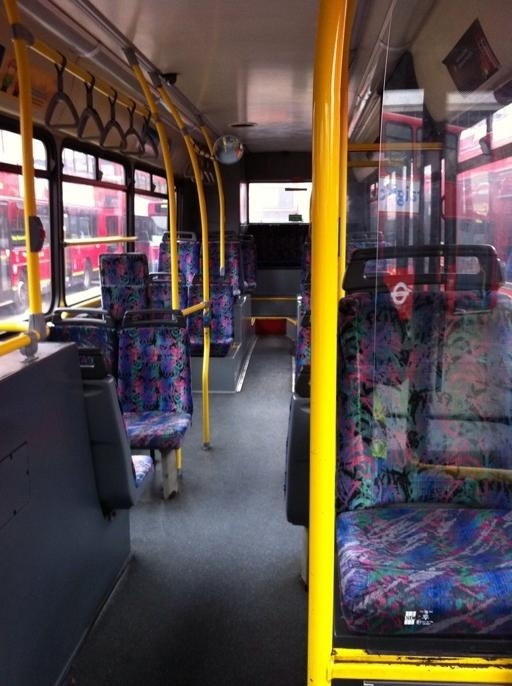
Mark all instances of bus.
[379,112,492,329]
[95,183,123,257]
[378,111,511,296]
[0,196,99,311]
[134,215,147,254]
[147,201,169,266]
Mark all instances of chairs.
[336,292,512,648]
[298,231,396,395]
[115,307,192,498]
[48,307,116,380]
[98,224,259,355]
